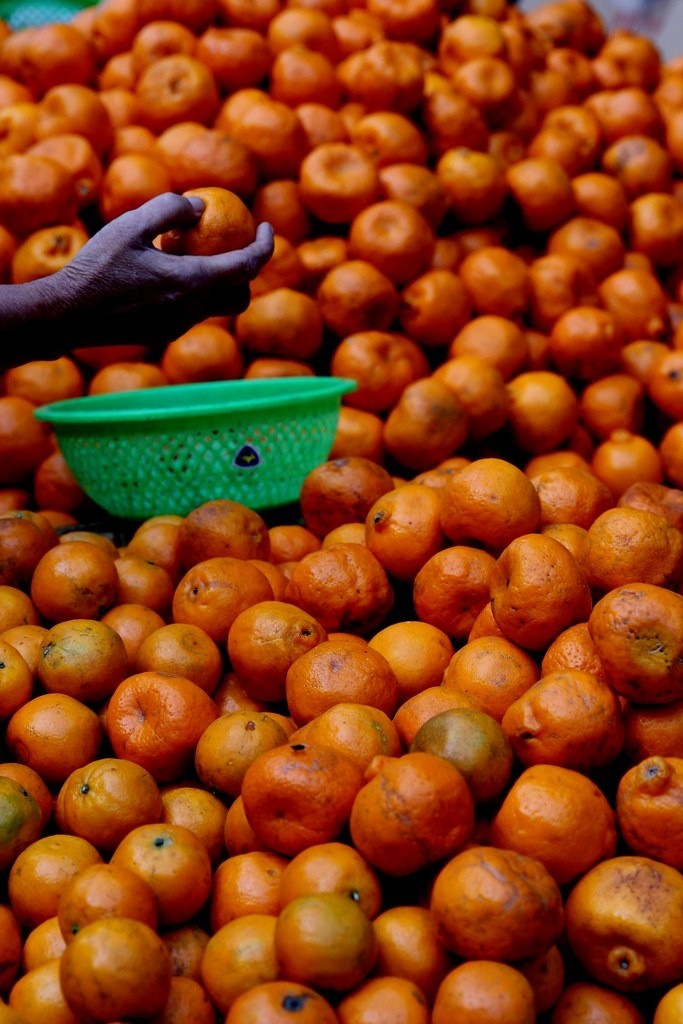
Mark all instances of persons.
[0,193,276,381]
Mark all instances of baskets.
[35,374,355,516]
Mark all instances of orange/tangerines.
[0,0,683,1024]
